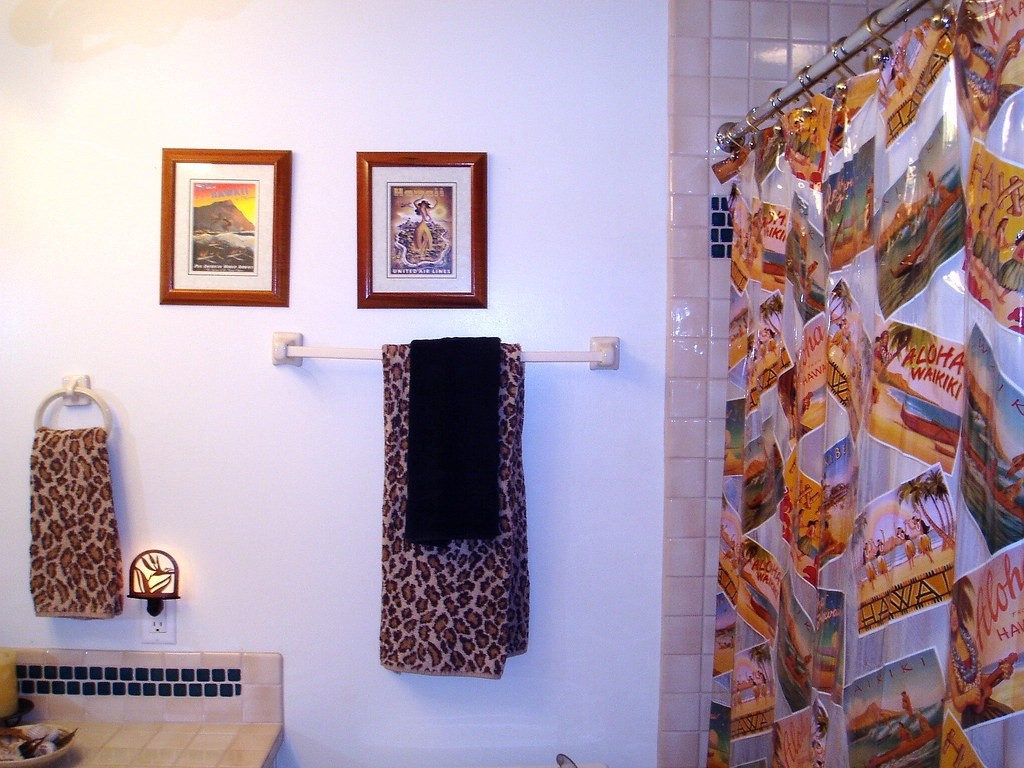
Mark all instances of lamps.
[126,550,181,616]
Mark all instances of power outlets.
[142,599,176,644]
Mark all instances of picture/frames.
[160,148,292,307]
[357,151,487,309]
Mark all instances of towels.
[27,425,127,622]
[377,335,532,681]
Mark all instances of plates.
[0,725,75,768]
